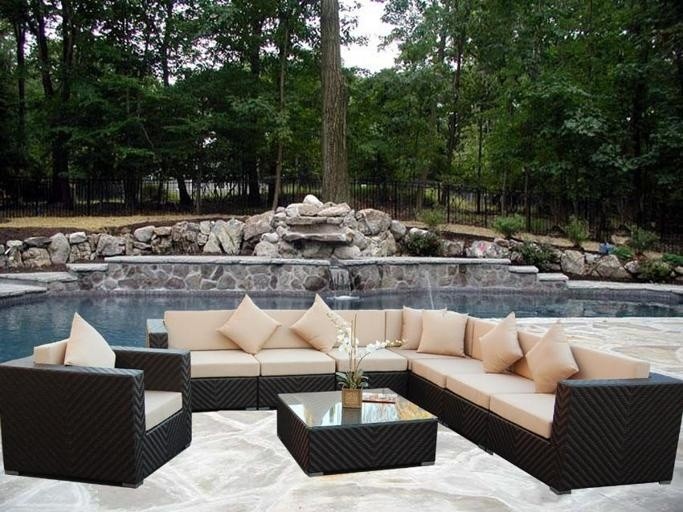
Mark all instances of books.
[362,393,397,403]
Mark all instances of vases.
[340,387,363,409]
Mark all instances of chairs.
[0,345,193,489]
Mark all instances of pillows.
[63,311,118,370]
[400,305,448,351]
[218,293,283,357]
[477,312,523,374]
[420,314,468,359]
[527,319,580,394]
[289,293,350,354]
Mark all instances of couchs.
[147,318,683,495]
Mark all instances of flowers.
[314,303,408,390]
